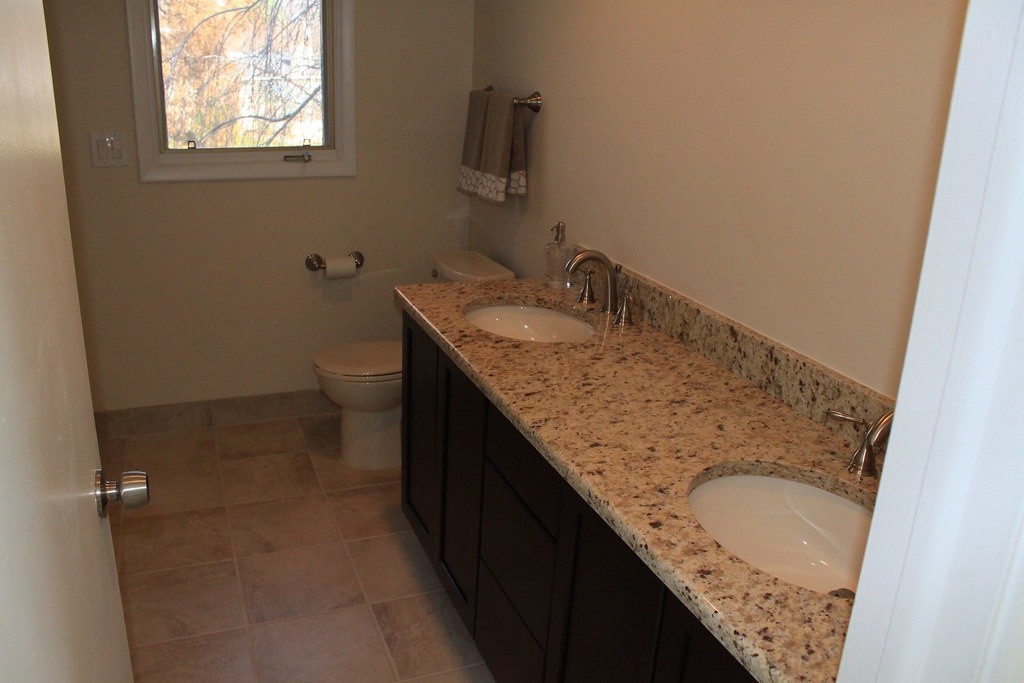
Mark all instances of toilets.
[311,249,516,469]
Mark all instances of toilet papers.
[324,255,356,278]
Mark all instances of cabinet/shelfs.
[389,247,899,683]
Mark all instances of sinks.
[687,459,877,600]
[459,295,596,343]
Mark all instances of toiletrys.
[537,221,578,290]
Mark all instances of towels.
[456,90,528,206]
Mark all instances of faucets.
[564,250,618,313]
[866,409,893,446]
